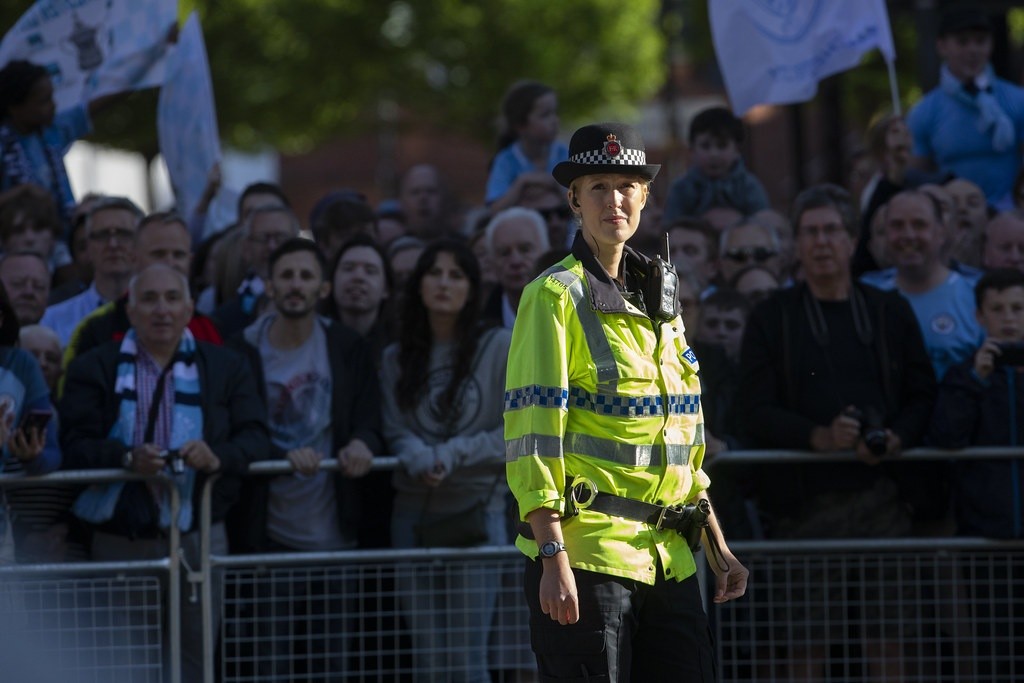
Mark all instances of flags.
[157,11,222,229]
[707,0,897,116]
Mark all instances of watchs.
[538,539,566,560]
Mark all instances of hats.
[552,123,662,189]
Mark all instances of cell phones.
[14,410,52,447]
[991,342,1024,366]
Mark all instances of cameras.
[160,449,186,473]
[850,411,888,456]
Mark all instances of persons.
[627,12,1024,683]
[0,58,657,683]
[504,122,752,683]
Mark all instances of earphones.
[572,197,580,207]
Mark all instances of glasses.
[722,245,778,264]
[89,227,133,242]
